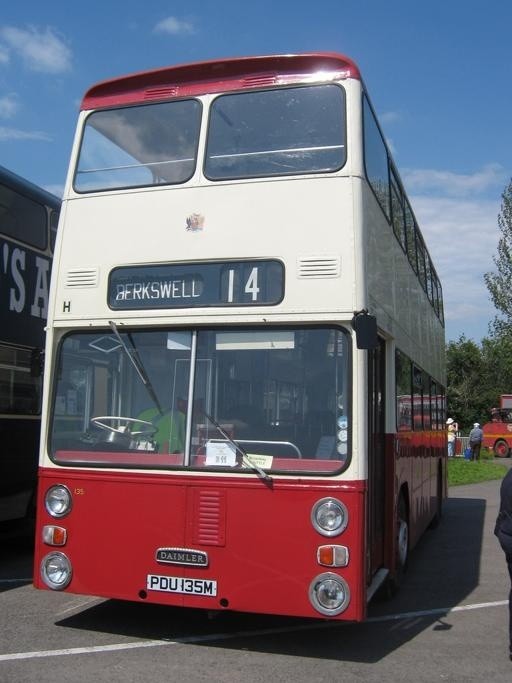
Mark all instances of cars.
[481,394,512,458]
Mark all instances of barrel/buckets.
[464,447,471,458]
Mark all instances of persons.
[130,398,187,452]
[469,422,484,461]
[446,417,458,457]
[494,467,512,661]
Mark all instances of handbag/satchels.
[464,447,472,460]
[448,434,456,442]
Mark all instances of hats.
[473,422,480,425]
[445,417,454,424]
